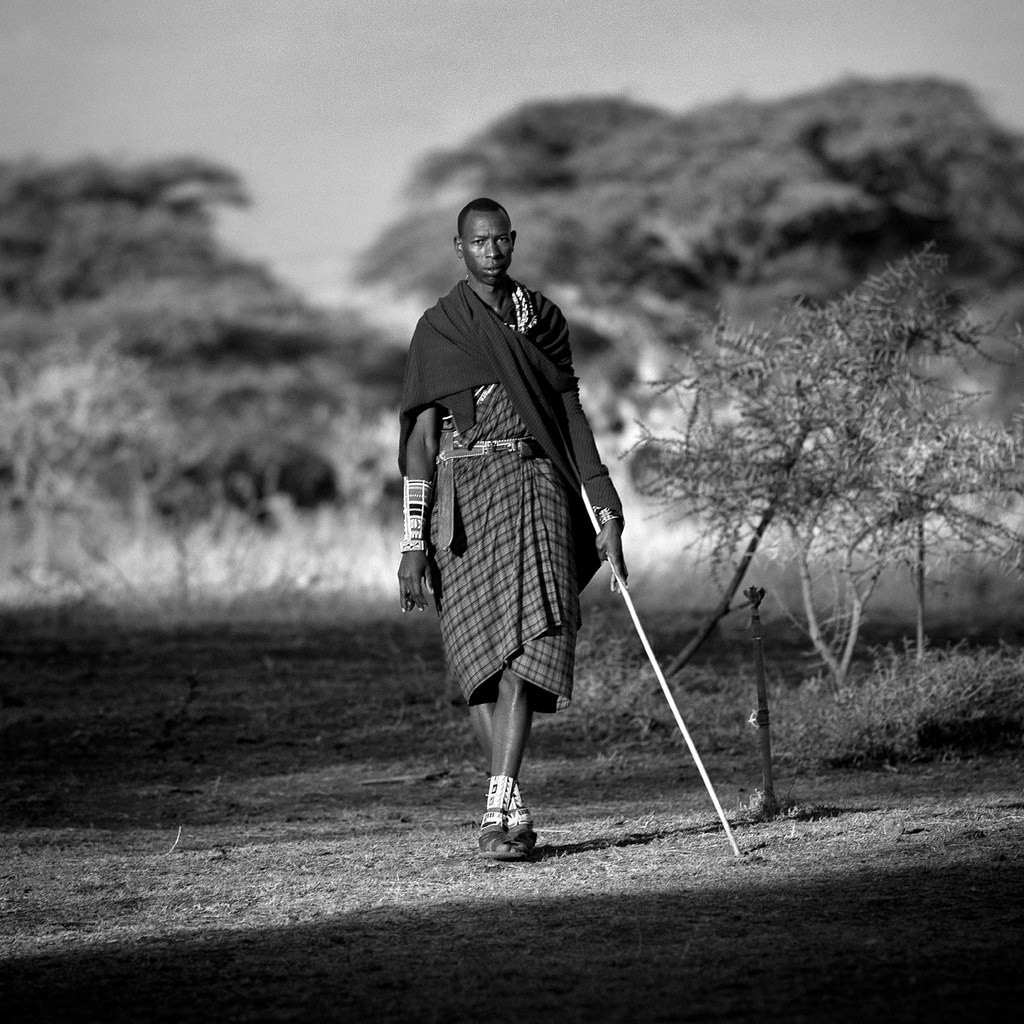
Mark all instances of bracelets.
[596,507,620,525]
[400,540,430,558]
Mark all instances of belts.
[439,438,538,461]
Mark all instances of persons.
[398,196,629,859]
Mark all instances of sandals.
[501,830,537,852]
[478,827,526,859]
